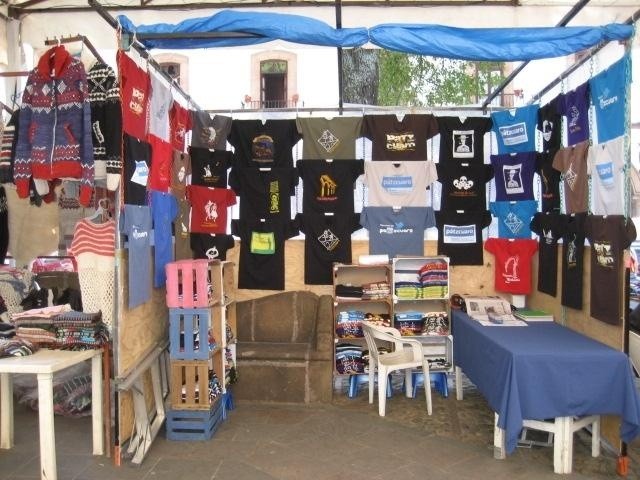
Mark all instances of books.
[464,298,554,328]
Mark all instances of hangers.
[85,199,113,224]
[47,38,64,59]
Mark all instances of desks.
[449,307,629,478]
[0,347,103,480]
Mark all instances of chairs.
[359,318,434,417]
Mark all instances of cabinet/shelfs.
[332,256,455,399]
[164,257,239,441]
